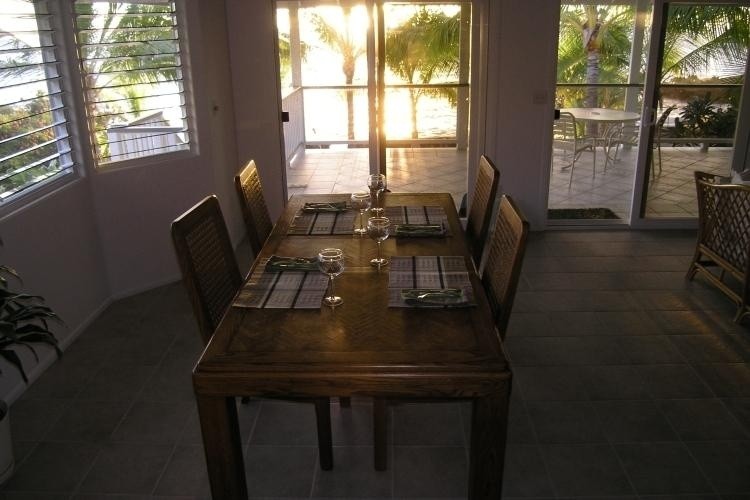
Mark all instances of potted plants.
[0,264,69,486]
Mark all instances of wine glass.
[317,248,345,306]
[367,173,386,213]
[366,216,391,267]
[351,191,371,236]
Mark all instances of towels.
[400,287,468,306]
[302,200,350,214]
[394,223,445,238]
[264,255,322,271]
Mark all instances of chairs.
[370,193,534,473]
[462,152,502,270]
[231,157,273,259]
[169,192,353,471]
[685,170,750,324]
[552,106,674,190]
